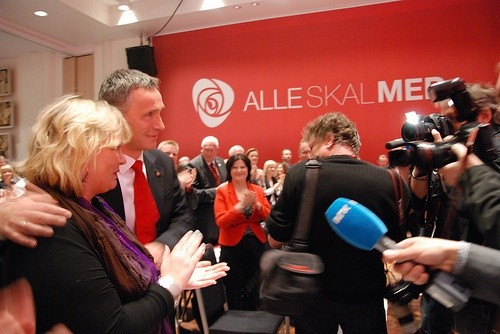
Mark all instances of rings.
[20,221,26,232]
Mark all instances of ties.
[129,160,160,245]
[209,163,221,187]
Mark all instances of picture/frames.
[0,68,11,96]
[0,132,13,162]
[0,99,14,129]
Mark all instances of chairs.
[194,244,292,334]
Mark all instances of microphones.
[385,138,406,150]
[323,197,471,312]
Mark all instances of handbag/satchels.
[259,247,324,318]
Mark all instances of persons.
[266,110,410,334]
[379,81,500,334]
[0,68,311,334]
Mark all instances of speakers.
[125,45,157,77]
